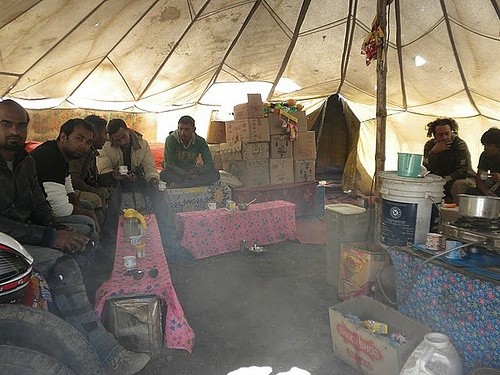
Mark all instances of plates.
[249,246,268,252]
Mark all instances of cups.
[118,165,128,175]
[135,242,145,259]
[445,240,462,259]
[158,182,167,191]
[425,233,442,251]
[123,256,136,271]
[208,203,216,211]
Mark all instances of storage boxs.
[329,296,431,375]
[205,92,317,188]
[337,241,396,301]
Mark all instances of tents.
[0,0,500,177]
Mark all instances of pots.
[456,181,500,220]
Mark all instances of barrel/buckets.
[397,152,423,177]
[399,332,463,375]
[473,368,500,375]
[377,170,447,250]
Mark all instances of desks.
[173,199,296,258]
[94,214,194,355]
[233,180,317,217]
[388,244,500,375]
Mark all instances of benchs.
[167,184,233,210]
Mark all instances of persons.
[0,98,150,375]
[472,128,500,196]
[160,116,220,189]
[96,119,170,237]
[423,118,477,204]
[69,115,127,242]
[30,119,100,288]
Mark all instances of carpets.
[294,220,326,245]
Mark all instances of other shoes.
[103,348,151,375]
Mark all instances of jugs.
[122,208,147,242]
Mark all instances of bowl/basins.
[238,203,249,211]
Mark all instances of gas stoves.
[436,217,500,254]
